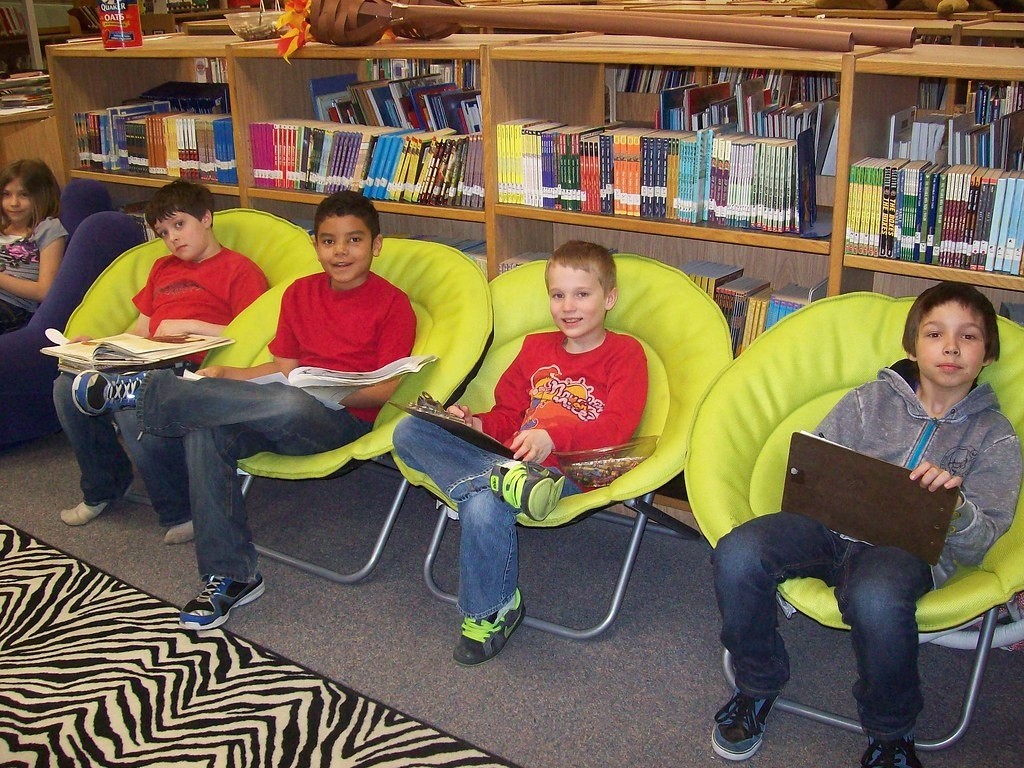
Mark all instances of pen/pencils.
[819,432,826,439]
[457,403,460,409]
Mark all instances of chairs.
[390,254,734,640]
[56,207,318,505]
[683,291,1024,748]
[195,239,493,585]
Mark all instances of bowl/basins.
[552,435,660,487]
[222,11,286,42]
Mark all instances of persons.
[392,241,648,666]
[712,281,1022,768]
[53,180,270,543]
[0,159,69,334]
[73,191,416,631]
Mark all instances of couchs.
[0,179,144,449]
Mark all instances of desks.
[1,110,65,191]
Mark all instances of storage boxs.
[98,0,142,49]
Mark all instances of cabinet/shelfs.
[0,0,1024,537]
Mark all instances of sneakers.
[179,573,266,630]
[453,588,525,665]
[72,370,146,416]
[711,688,781,761]
[490,462,565,522]
[861,724,923,768]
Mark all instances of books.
[0,71,53,109]
[67,4,101,32]
[0,7,26,32]
[40,58,1024,411]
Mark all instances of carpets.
[0,520,516,767]
